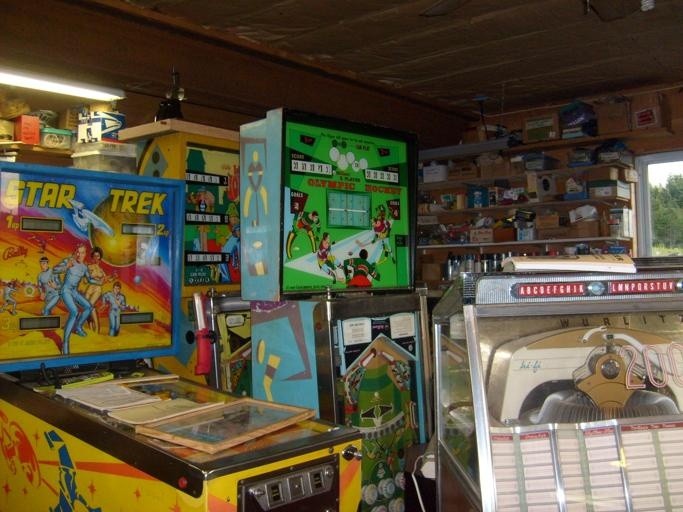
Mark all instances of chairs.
[0,94,139,176]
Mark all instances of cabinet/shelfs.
[416,129,674,285]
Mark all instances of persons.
[99,281,125,337]
[53,244,104,356]
[342,249,380,288]
[316,231,342,284]
[370,218,395,264]
[37,256,62,315]
[0,282,18,315]
[83,246,117,334]
[286,210,321,258]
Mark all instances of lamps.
[641,0,654,11]
[0,67,128,101]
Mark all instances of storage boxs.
[421,263,444,280]
[423,91,671,244]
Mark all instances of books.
[561,130,588,140]
[561,127,584,133]
[108,397,222,425]
[54,381,160,412]
[499,252,638,274]
